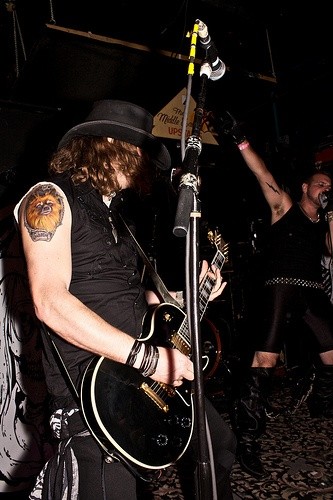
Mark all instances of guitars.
[78,225,231,475]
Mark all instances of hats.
[57,100,171,170]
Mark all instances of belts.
[265,277,324,290]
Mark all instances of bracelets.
[125,339,160,379]
[239,143,250,151]
[175,291,184,308]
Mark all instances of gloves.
[207,109,245,145]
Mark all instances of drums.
[199,315,222,381]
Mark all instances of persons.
[12,98,228,500]
[215,110,333,479]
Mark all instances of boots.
[231,372,269,479]
[309,365,333,418]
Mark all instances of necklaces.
[304,213,321,225]
[103,210,120,245]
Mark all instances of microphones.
[195,19,226,81]
